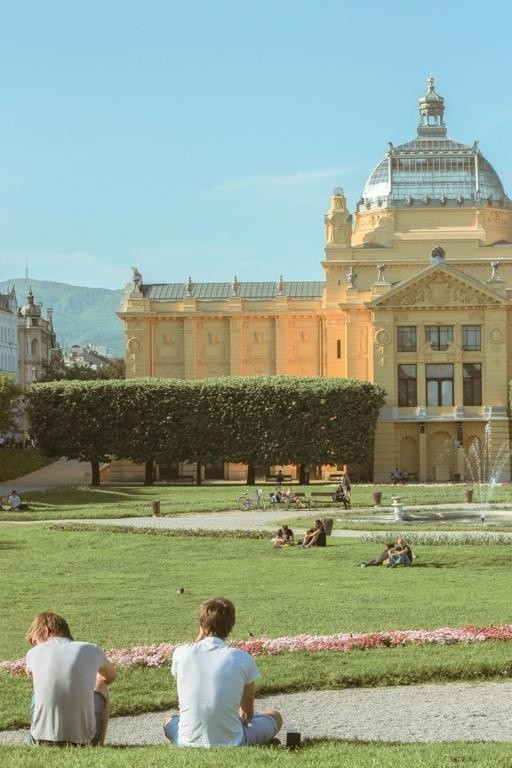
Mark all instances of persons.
[392,467,401,484]
[298,519,326,548]
[341,472,351,495]
[9,490,31,511]
[24,611,118,747]
[401,468,409,485]
[272,524,294,549]
[385,537,412,567]
[274,486,290,511]
[335,484,351,509]
[352,543,395,568]
[163,597,283,749]
[286,486,307,511]
[276,469,283,485]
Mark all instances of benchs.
[266,491,313,511]
[165,474,195,486]
[330,473,343,481]
[263,474,293,485]
[390,471,419,484]
[309,490,350,509]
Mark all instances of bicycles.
[237,488,268,512]
